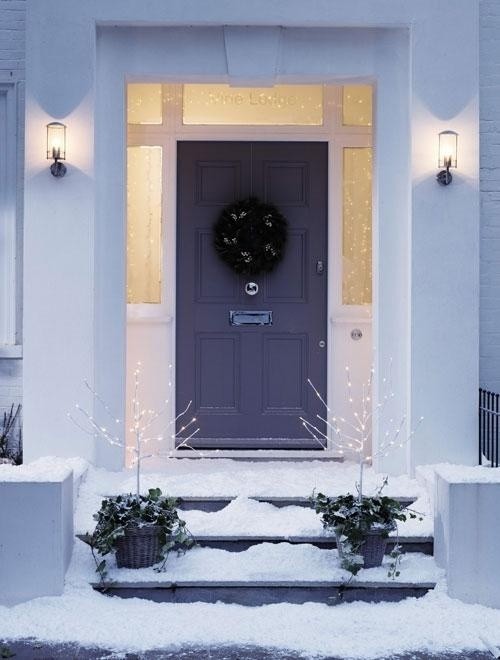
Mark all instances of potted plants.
[63,355,201,601]
[289,348,424,606]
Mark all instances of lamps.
[434,126,462,187]
[43,116,67,178]
